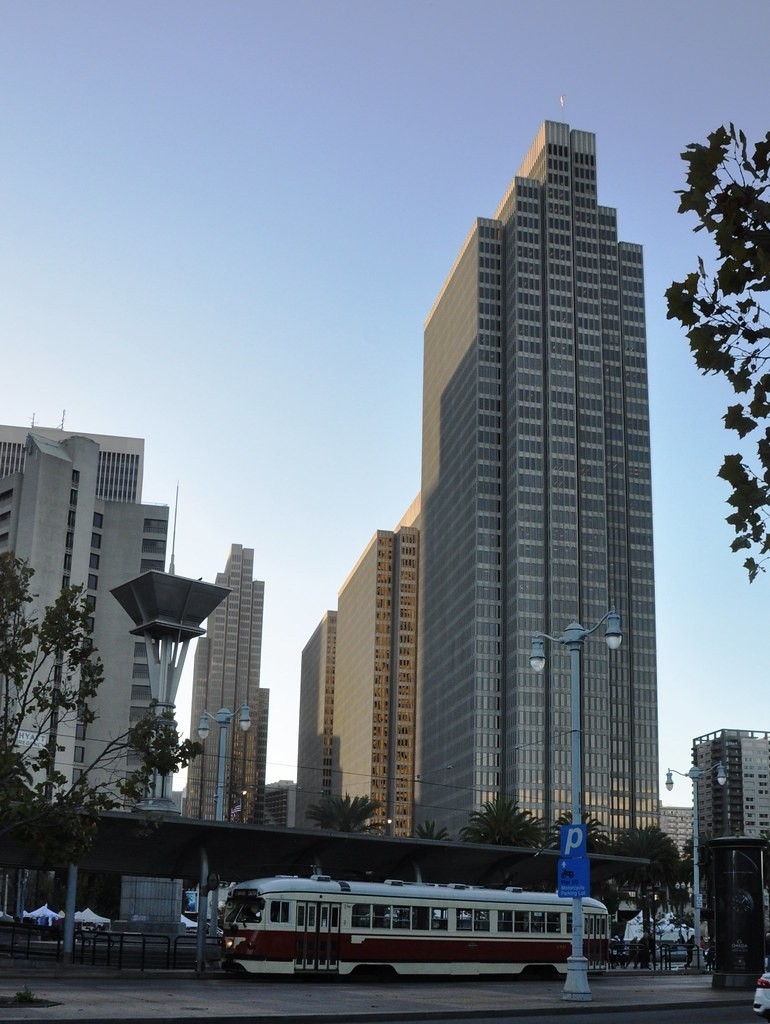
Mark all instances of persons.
[609,932,716,970]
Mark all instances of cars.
[752,971,770,1019]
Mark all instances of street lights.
[675,882,686,922]
[528,605,623,1002]
[665,761,728,973]
[198,700,252,966]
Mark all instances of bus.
[221,876,610,983]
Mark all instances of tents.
[181,914,198,928]
[0,905,111,932]
